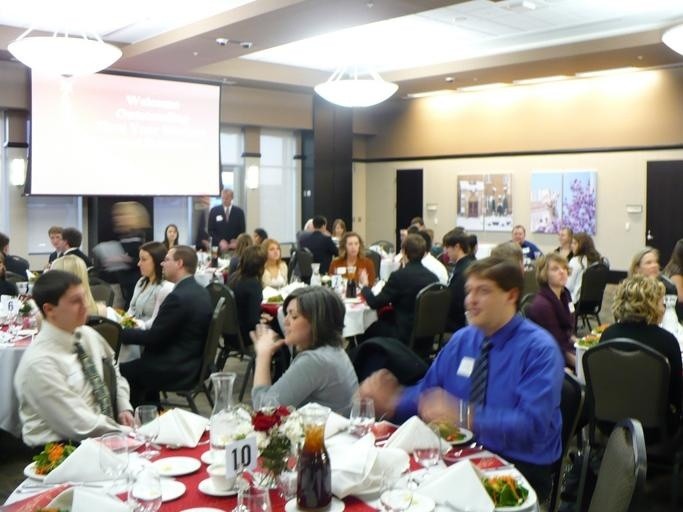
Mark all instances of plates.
[574,337,589,350]
[200,449,261,464]
[198,477,246,495]
[591,330,602,336]
[496,486,537,512]
[380,490,435,512]
[24,461,49,481]
[285,493,346,512]
[439,428,473,445]
[131,480,186,501]
[151,456,199,476]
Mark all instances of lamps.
[9,158,27,187]
[314,39,399,107]
[8,10,123,76]
[244,164,261,190]
[661,22,683,57]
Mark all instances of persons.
[1,189,682,511]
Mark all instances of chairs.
[538,367,588,512]
[587,417,647,512]
[581,338,683,505]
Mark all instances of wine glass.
[16,282,28,295]
[98,397,440,507]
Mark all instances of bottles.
[662,294,678,336]
[310,263,321,286]
[210,371,243,452]
[321,272,331,288]
[297,405,334,506]
[346,266,356,297]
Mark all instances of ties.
[225,208,230,223]
[73,340,114,419]
[468,338,493,408]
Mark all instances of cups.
[206,464,242,490]
[237,485,272,512]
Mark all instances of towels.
[328,434,411,499]
[48,441,137,479]
[138,409,210,448]
[383,416,452,451]
[46,487,134,511]
[418,461,495,512]
[323,412,350,440]
